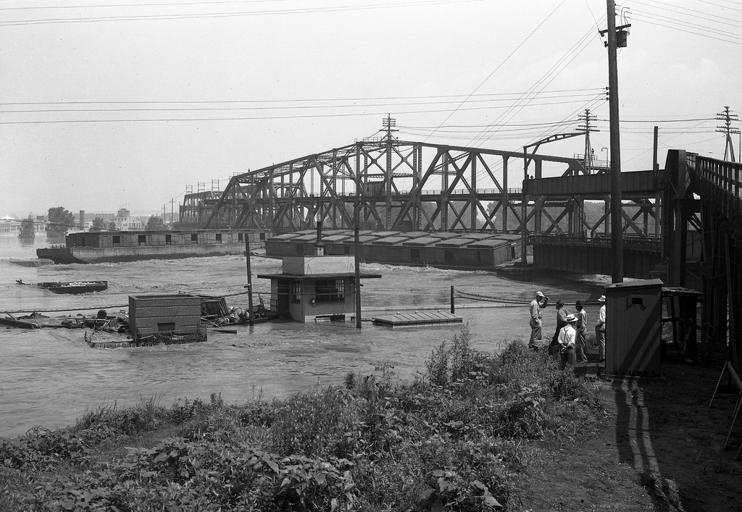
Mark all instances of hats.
[536,291,545,297]
[598,295,606,302]
[564,314,578,323]
[575,300,583,307]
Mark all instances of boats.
[15,279,109,296]
[35,226,278,263]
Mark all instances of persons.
[551,299,570,351]
[529,290,549,351]
[595,295,606,361]
[575,300,588,364]
[558,314,579,369]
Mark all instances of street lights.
[599,146,609,168]
